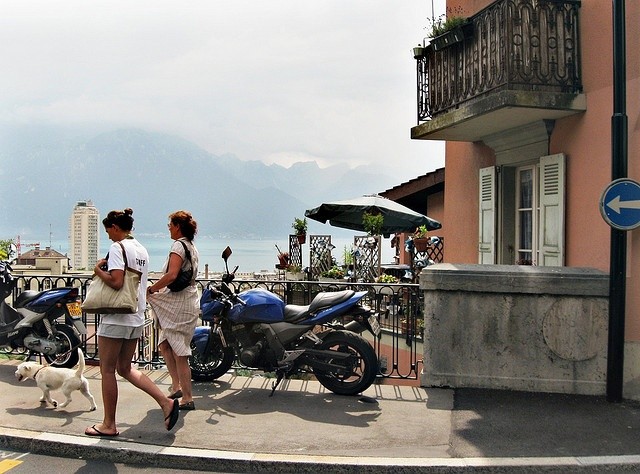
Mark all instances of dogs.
[14,347,96,411]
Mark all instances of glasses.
[168,223,176,227]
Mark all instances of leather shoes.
[178,401,194,410]
[167,390,183,399]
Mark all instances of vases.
[277,258,290,267]
[319,277,349,287]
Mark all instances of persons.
[146,210,201,411]
[84,207,180,437]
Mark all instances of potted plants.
[412,225,429,252]
[284,263,305,281]
[401,302,421,335]
[341,244,354,276]
[413,44,425,60]
[291,216,308,245]
[372,273,403,296]
[362,210,384,249]
[423,5,474,52]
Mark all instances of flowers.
[321,264,345,279]
[279,253,288,260]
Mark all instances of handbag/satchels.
[80,266,143,314]
[166,267,193,293]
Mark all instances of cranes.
[16,235,40,258]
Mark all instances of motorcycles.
[0,244,88,367]
[188,245,383,396]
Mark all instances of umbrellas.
[304,193,443,271]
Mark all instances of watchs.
[147,285,154,296]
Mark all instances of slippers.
[165,399,179,431]
[85,425,119,436]
